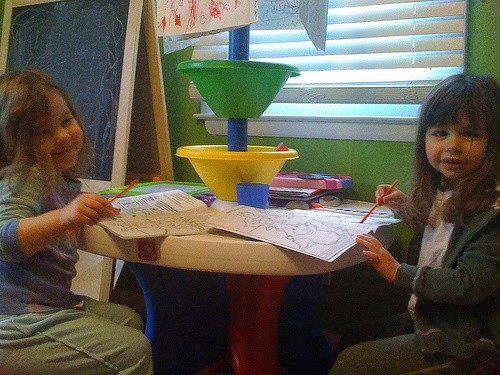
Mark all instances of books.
[96,189,232,240]
[269,172,352,207]
[266,186,344,202]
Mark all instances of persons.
[329,74,500,375]
[1,70,154,375]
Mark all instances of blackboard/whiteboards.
[0,0,145,195]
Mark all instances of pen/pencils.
[67,179,139,236]
[360,179,399,224]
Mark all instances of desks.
[77,195,397,375]
